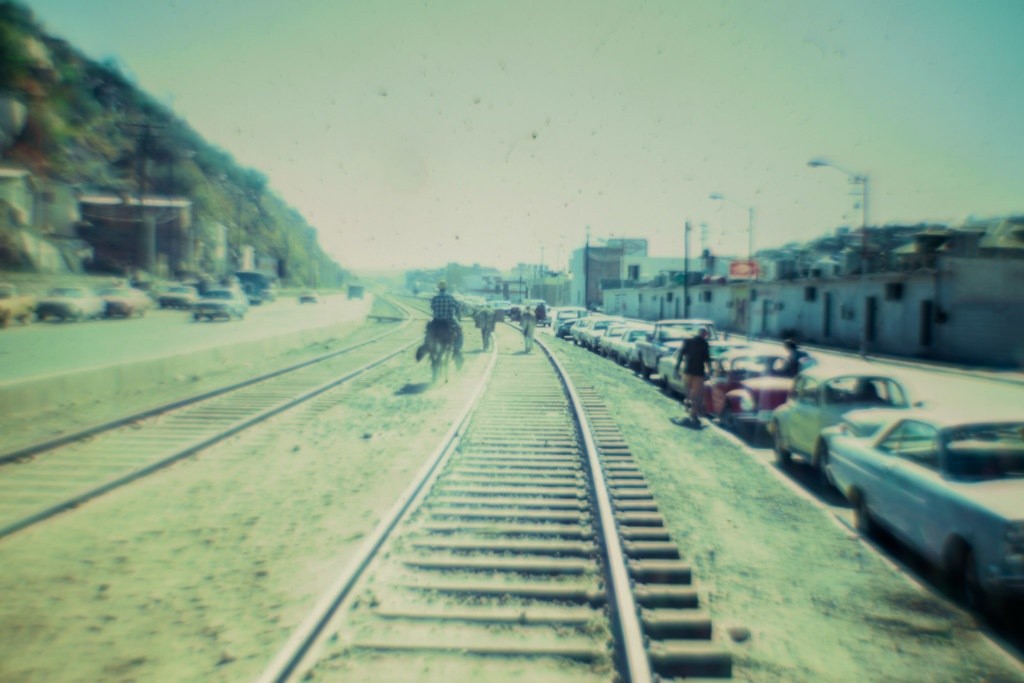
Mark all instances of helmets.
[437,279,448,290]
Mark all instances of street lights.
[710,193,752,339]
[807,159,865,356]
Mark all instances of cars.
[158,286,200,311]
[771,365,915,476]
[194,289,248,319]
[828,403,1024,604]
[37,288,106,323]
[487,300,818,428]
[301,289,320,303]
[98,287,151,319]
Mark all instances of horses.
[426,321,458,384]
[468,307,495,351]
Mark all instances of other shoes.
[688,415,699,427]
[452,349,461,357]
[525,348,531,355]
[432,348,437,355]
[483,346,489,352]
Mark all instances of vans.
[234,272,267,303]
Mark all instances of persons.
[778,339,801,378]
[673,326,711,430]
[430,280,464,355]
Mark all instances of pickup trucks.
[0,282,36,328]
